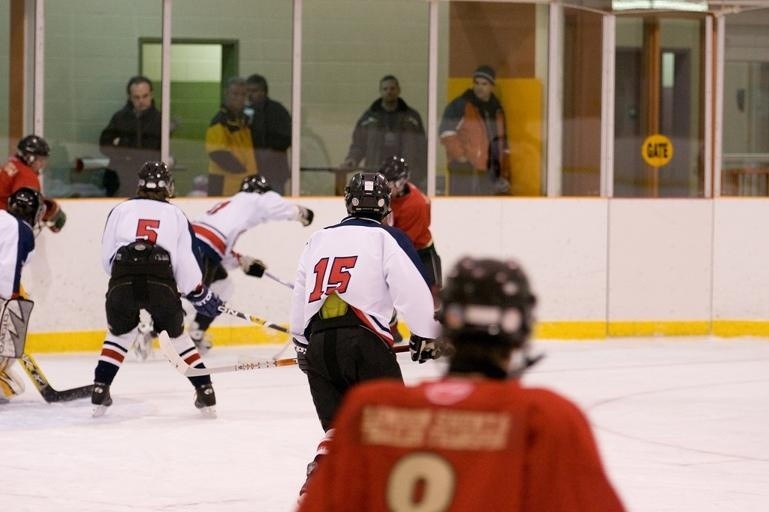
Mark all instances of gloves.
[43,198,67,234]
[296,345,308,374]
[239,255,265,278]
[409,334,441,364]
[187,284,223,318]
[298,204,313,227]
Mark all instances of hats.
[474,65,496,84]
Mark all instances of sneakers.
[195,385,216,408]
[91,382,114,407]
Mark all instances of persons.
[93,162,215,407]
[0,135,66,233]
[439,66,509,195]
[0,187,40,401]
[137,175,313,352]
[207,76,258,197]
[379,156,442,313]
[296,259,627,512]
[101,77,161,196]
[291,171,443,506]
[244,75,292,196]
[342,75,428,196]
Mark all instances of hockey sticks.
[20,350,94,404]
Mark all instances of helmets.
[18,135,49,157]
[138,161,170,192]
[345,172,390,219]
[436,259,536,355]
[8,187,40,228]
[377,154,409,179]
[241,175,271,193]
